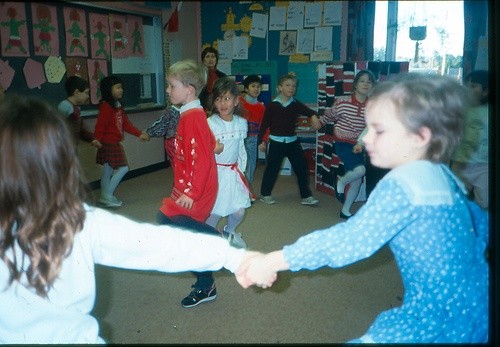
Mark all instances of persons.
[198,47,227,119]
[311,69,378,220]
[142,104,182,171]
[257,74,320,205]
[236,68,488,343]
[448,70,488,211]
[207,77,252,251]
[0,95,277,343]
[56,75,102,155]
[236,74,270,191]
[157,58,248,308]
[94,76,150,208]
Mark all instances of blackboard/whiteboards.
[0,0,167,114]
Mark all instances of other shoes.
[335,180,345,204]
[250,194,255,201]
[339,209,354,219]
[96,196,123,208]
[224,225,247,249]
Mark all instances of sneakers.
[181,282,216,308]
[300,196,319,205]
[260,196,276,205]
[221,230,234,245]
